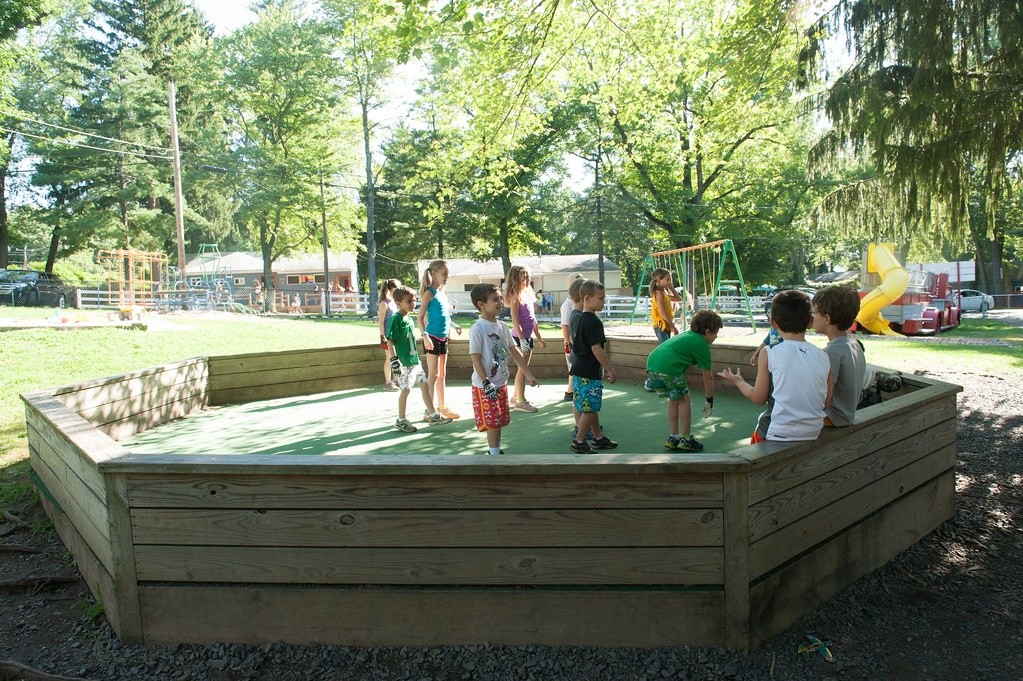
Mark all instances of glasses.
[812,311,820,317]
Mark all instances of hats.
[567,272,587,288]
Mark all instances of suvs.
[765,287,819,318]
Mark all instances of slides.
[855,245,907,337]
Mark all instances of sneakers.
[382,382,399,392]
[423,409,448,421]
[513,399,538,413]
[586,424,603,432]
[676,433,703,451]
[436,407,460,418]
[394,419,417,432]
[392,379,404,390]
[571,425,594,438]
[509,397,532,406]
[569,439,597,454]
[665,435,680,450]
[429,412,452,426]
[590,436,618,450]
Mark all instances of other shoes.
[644,378,655,392]
[564,392,574,401]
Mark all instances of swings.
[653,243,721,314]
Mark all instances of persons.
[561,273,617,455]
[536,290,554,316]
[417,258,462,423]
[749,327,784,366]
[468,284,539,457]
[377,279,402,391]
[291,292,301,307]
[505,265,545,414]
[254,280,262,303]
[314,282,355,302]
[215,282,223,301]
[720,290,829,443]
[645,267,682,391]
[645,309,722,450]
[811,285,866,429]
[386,285,452,433]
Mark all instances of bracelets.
[422,332,428,337]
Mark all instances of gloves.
[390,355,405,380]
[564,343,571,354]
[482,376,501,401]
[702,396,713,419]
[520,338,531,355]
[380,335,388,350]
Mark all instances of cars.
[0,270,67,308]
[947,289,994,313]
